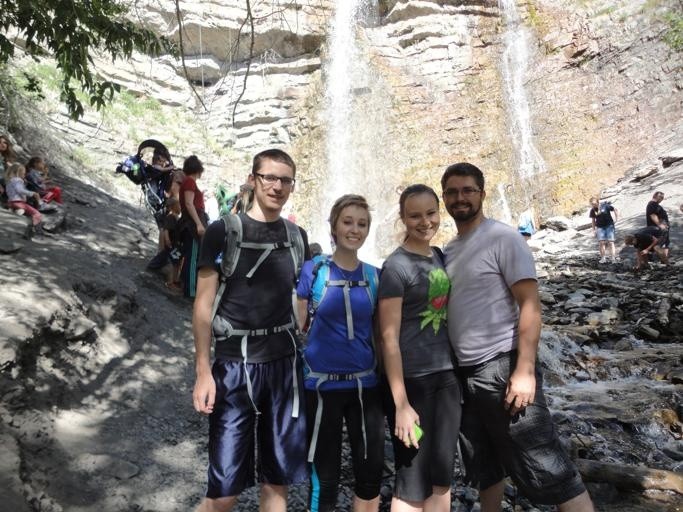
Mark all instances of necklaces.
[339,264,357,292]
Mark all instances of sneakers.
[140,273,152,279]
[165,282,181,293]
[33,229,43,235]
[612,259,618,264]
[599,259,607,264]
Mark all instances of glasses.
[255,173,294,185]
[442,188,483,196]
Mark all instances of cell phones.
[398,423,424,450]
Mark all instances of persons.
[25,157,64,204]
[514,203,536,242]
[190,148,311,511]
[5,161,57,239]
[376,184,464,511]
[295,192,382,511]
[145,151,253,301]
[589,197,618,264]
[645,190,671,250]
[440,162,596,511]
[0,134,10,205]
[623,223,669,271]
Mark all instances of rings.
[520,401,529,407]
